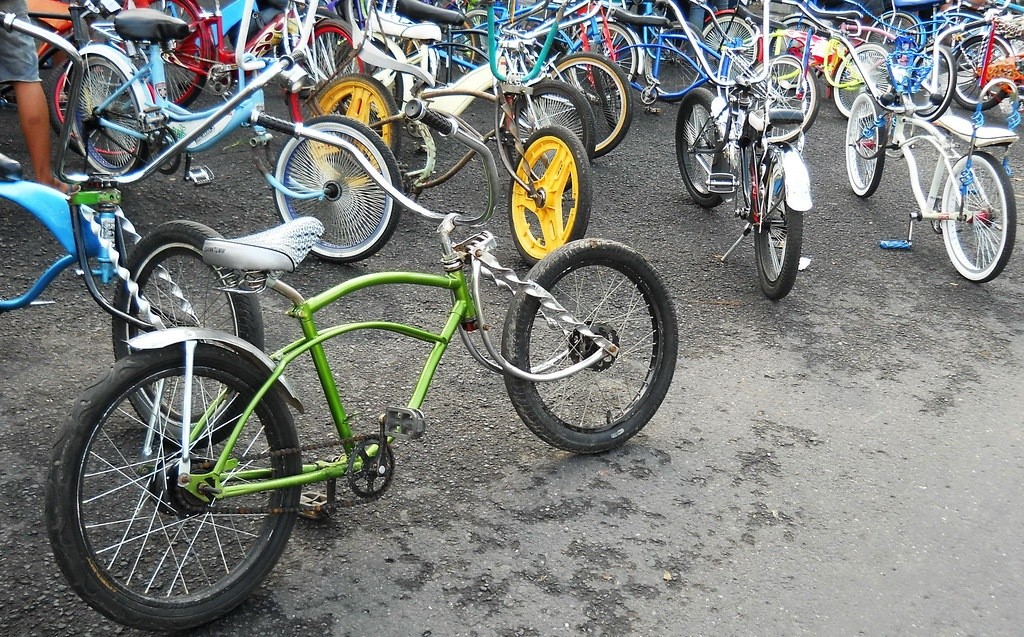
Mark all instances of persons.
[663,0,745,64]
[0,0,82,196]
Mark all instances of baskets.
[884,51,933,94]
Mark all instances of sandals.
[65,184,81,195]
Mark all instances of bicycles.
[0,0,1024,448]
[44,99,678,631]
[985,230,990,232]
